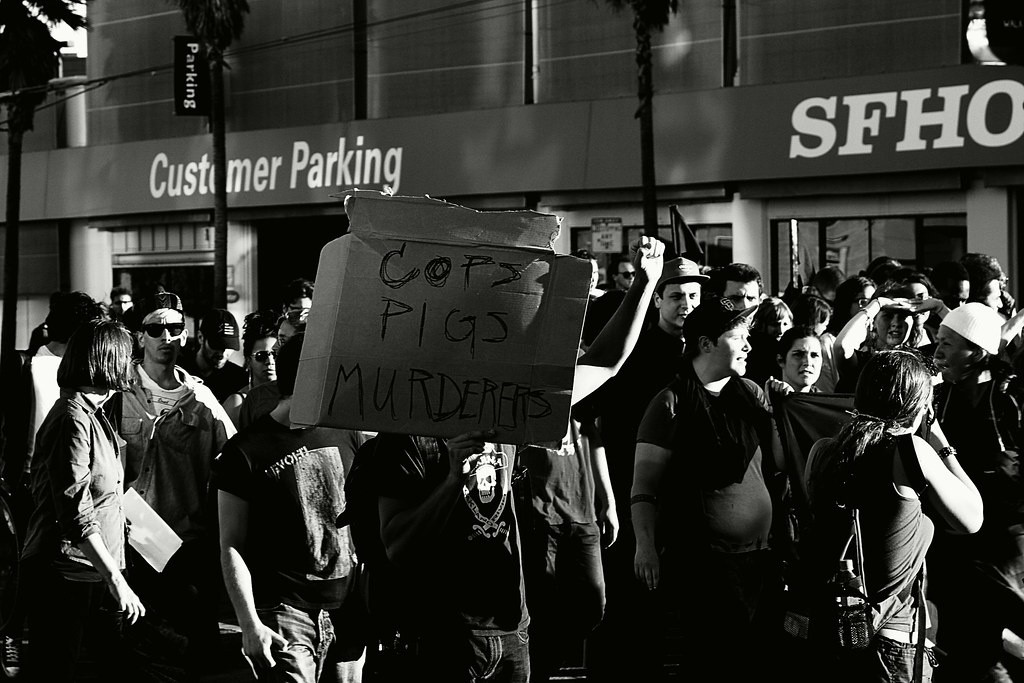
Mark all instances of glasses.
[143,322,186,338]
[250,350,276,364]
[620,271,638,279]
[852,297,869,309]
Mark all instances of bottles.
[836,559,868,647]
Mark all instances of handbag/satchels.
[773,568,873,651]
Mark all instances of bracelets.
[861,308,872,327]
[938,447,958,458]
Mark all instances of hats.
[940,302,1001,355]
[656,257,711,290]
[139,292,184,322]
[682,296,760,360]
[201,309,241,349]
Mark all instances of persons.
[1,232,1024,682]
